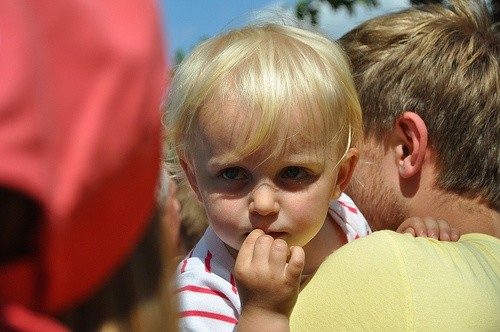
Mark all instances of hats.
[1,1,165,330]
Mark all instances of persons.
[169,8,460,332]
[289,0,500,332]
[0,0,210,332]
